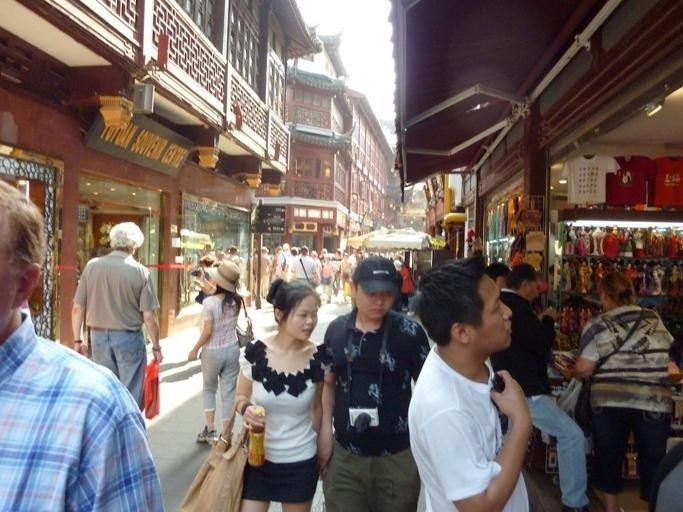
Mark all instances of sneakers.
[197,427,218,443]
[219,432,231,449]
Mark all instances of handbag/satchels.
[321,276,331,285]
[575,377,593,437]
[144,357,160,419]
[542,377,594,454]
[236,317,254,347]
[309,280,319,288]
[180,397,252,512]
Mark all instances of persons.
[407,257,530,510]
[236,275,324,512]
[70,221,163,413]
[322,254,430,511]
[189,261,241,445]
[189,245,416,313]
[485,264,682,510]
[0,177,165,512]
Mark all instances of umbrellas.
[350,227,437,253]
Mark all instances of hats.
[203,261,240,293]
[352,256,402,294]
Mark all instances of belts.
[89,328,143,333]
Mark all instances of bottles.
[247,406,267,468]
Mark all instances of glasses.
[493,374,508,435]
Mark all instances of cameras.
[348,407,381,434]
[190,270,202,278]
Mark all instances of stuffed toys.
[563,223,683,259]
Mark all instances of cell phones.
[492,374,504,392]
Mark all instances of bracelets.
[152,345,161,351]
[72,339,82,344]
